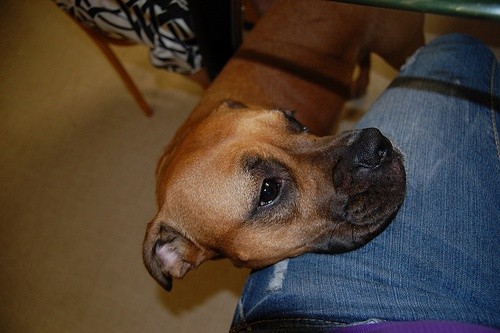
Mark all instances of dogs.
[141,0,426,293]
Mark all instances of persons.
[229,34,500,333]
[48,0,243,81]
